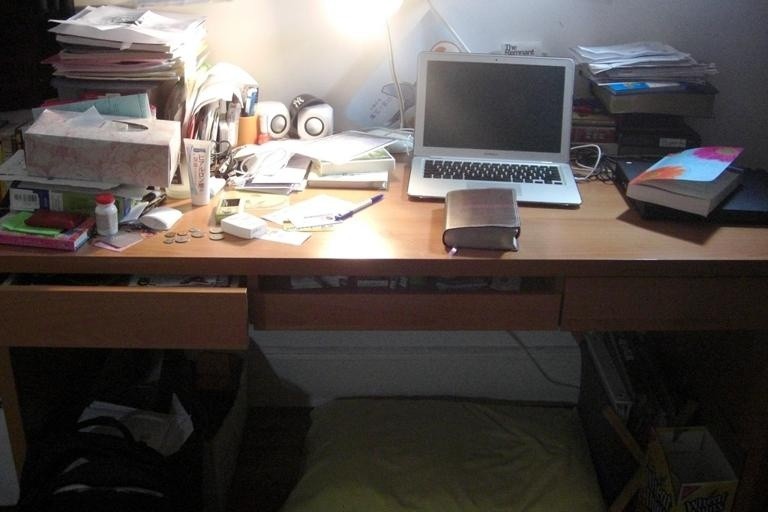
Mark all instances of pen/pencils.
[335,194,383,220]
[244,87,258,117]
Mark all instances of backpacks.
[19,417,174,512]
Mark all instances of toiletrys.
[182,136,212,205]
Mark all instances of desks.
[0,150,768,512]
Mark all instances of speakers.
[253,101,290,140]
[297,104,333,140]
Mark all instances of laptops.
[408,52,582,206]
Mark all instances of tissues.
[24,107,182,188]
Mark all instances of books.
[569,40,722,121]
[626,146,750,220]
[245,126,397,197]
[0,211,95,252]
[2,4,258,158]
[442,187,521,252]
